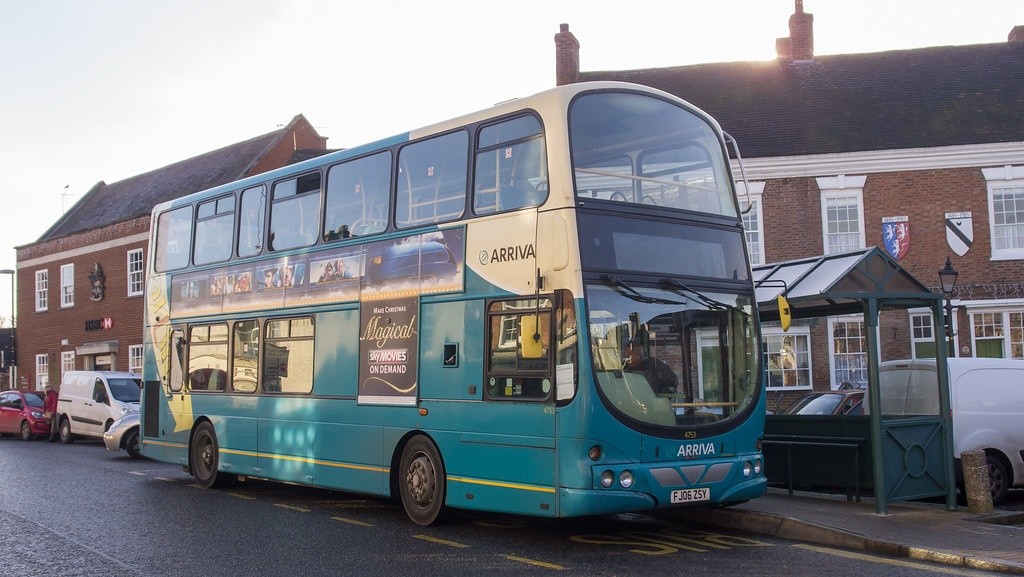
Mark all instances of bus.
[139,80,791,527]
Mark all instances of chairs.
[207,369,226,391]
[499,177,659,213]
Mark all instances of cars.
[104,409,139,459]
[0,388,58,441]
[778,379,866,415]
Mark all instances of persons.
[264,268,278,289]
[317,260,351,281]
[279,267,293,287]
[625,344,679,400]
[43,384,58,442]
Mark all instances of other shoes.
[47,438,55,442]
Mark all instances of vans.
[863,357,1024,506]
[55,371,142,443]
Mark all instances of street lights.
[0,270,15,388]
[939,256,958,358]
